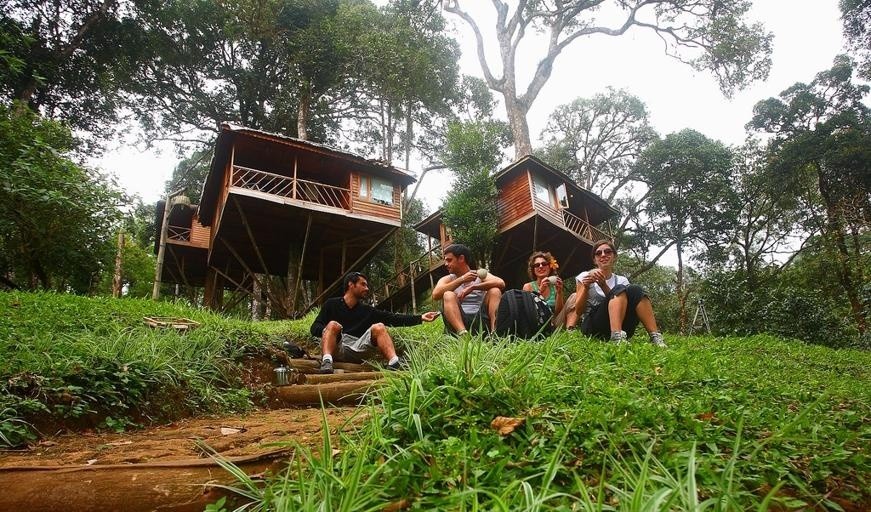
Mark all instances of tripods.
[687,304,712,338]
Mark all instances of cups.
[474,268,487,279]
[548,276,558,285]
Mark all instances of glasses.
[595,249,613,255]
[533,262,549,267]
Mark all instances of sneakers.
[320,359,334,373]
[610,331,627,343]
[387,357,404,370]
[649,333,668,347]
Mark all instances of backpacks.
[495,289,551,341]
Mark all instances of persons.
[431,244,507,339]
[120,278,129,297]
[311,271,442,372]
[576,240,669,349]
[523,251,580,333]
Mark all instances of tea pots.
[273,364,298,387]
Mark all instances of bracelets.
[461,275,465,281]
[538,287,544,293]
